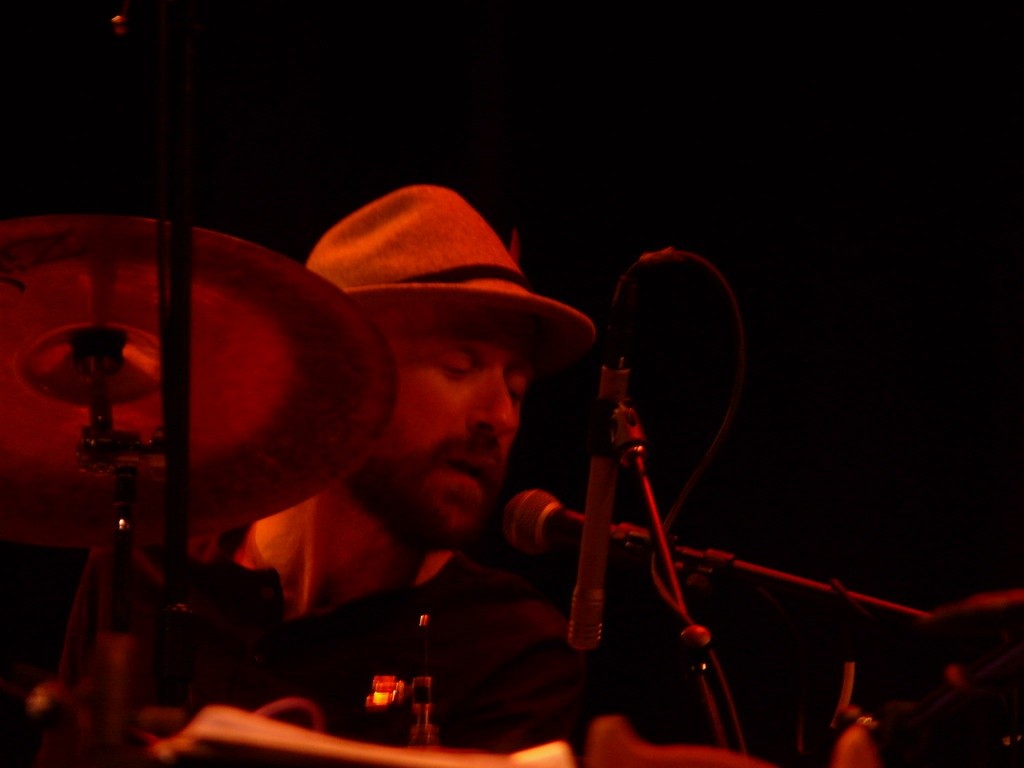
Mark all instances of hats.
[304,184,594,370]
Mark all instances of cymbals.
[0,201,410,559]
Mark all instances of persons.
[36,184,879,768]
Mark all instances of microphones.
[569,355,630,650]
[411,612,440,750]
[503,489,687,572]
